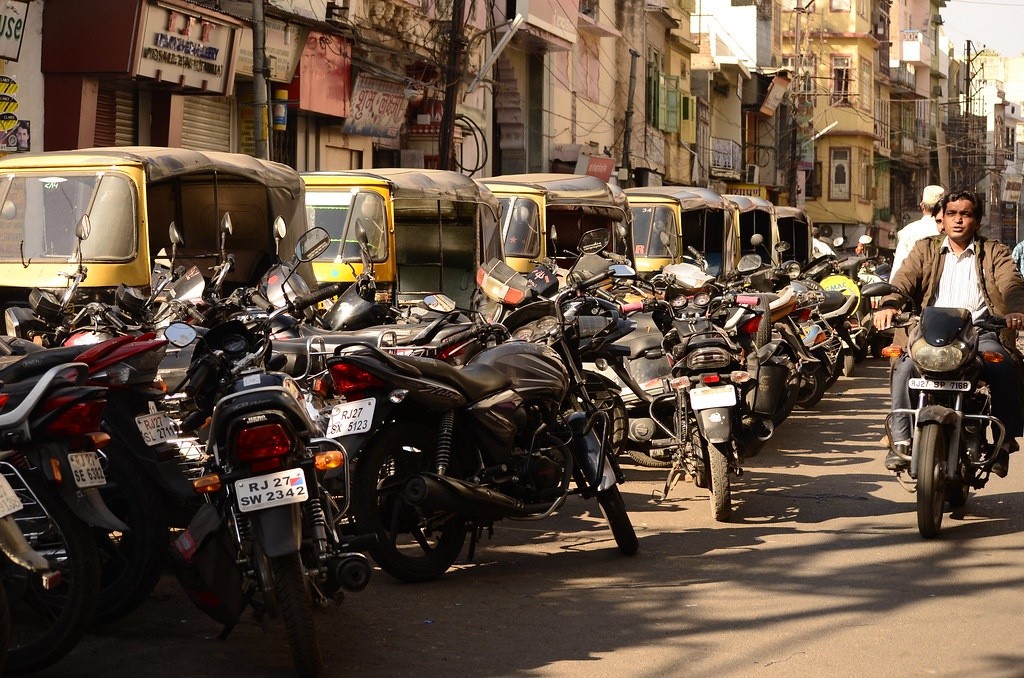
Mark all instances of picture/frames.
[828,147,852,202]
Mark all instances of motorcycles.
[0,212,896,678]
[0,146,813,335]
[861,282,1024,539]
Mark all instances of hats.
[923,185,944,205]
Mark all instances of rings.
[1018,320,1021,322]
[1012,318,1017,320]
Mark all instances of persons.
[889,185,944,285]
[874,188,1024,478]
[812,227,838,269]
[856,235,872,257]
[1012,240,1024,276]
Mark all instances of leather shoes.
[884,438,913,470]
[987,446,1009,478]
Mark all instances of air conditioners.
[818,224,842,240]
[746,164,759,184]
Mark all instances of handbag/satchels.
[744,338,798,415]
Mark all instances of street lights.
[440,13,524,170]
[789,121,838,207]
